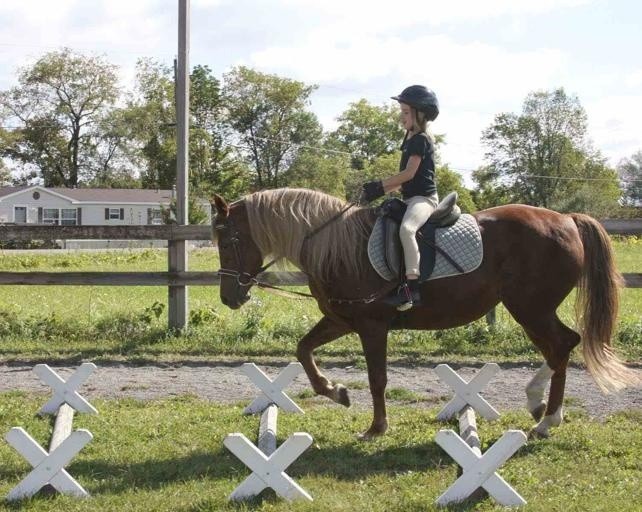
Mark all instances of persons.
[364,85,440,312]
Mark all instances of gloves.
[362,181,385,202]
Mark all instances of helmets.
[391,85,439,122]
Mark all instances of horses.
[209,187,640,443]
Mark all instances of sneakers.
[382,289,421,307]
